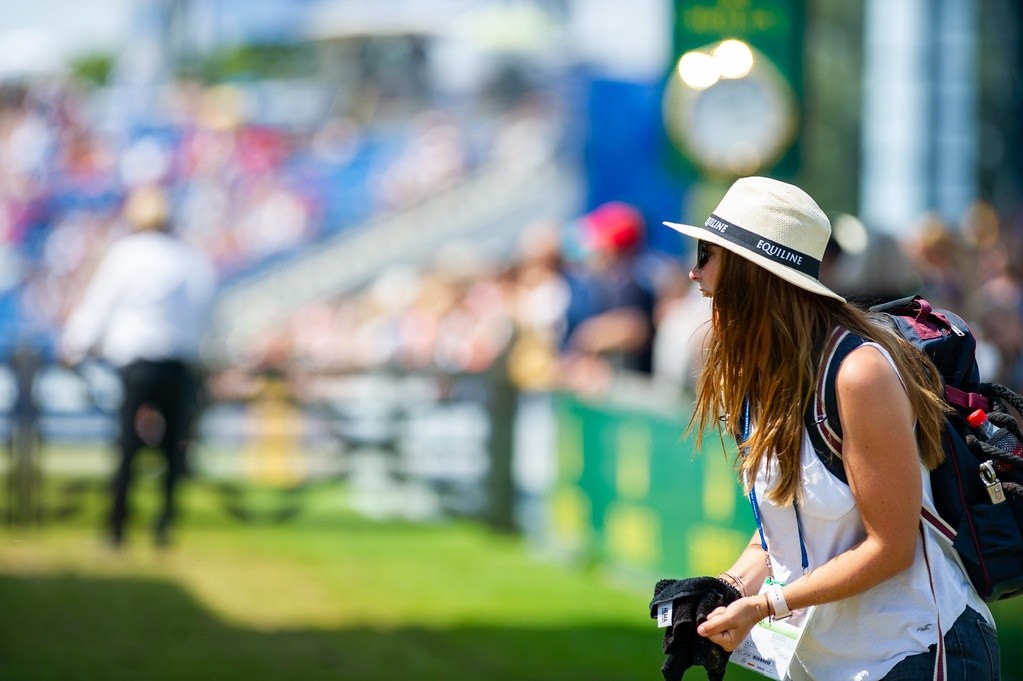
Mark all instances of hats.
[586,201,642,259]
[122,181,172,232]
[662,176,847,303]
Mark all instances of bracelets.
[763,592,771,627]
[722,571,748,596]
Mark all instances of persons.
[56,174,220,551]
[660,176,1001,681]
[247,199,1022,529]
[785,608,807,628]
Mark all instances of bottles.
[967,409,1023,474]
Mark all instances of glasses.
[697,239,721,270]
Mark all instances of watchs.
[769,586,793,621]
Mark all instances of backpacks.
[804,295,1023,603]
